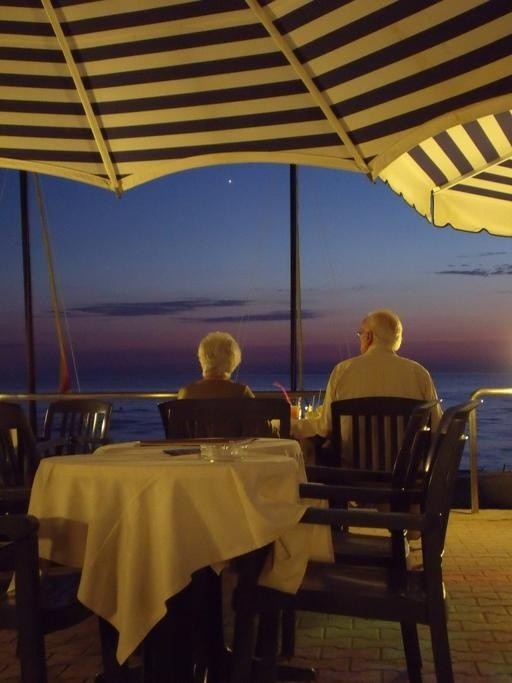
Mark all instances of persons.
[316,311,443,541]
[178,332,279,437]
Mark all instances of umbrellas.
[0,0,512,199]
[377,109,512,236]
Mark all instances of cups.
[290,397,302,420]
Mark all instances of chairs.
[153,401,295,436]
[101,433,314,659]
[2,400,129,681]
[41,400,116,458]
[229,401,480,680]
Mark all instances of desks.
[36,450,305,681]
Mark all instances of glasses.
[354,328,368,337]
